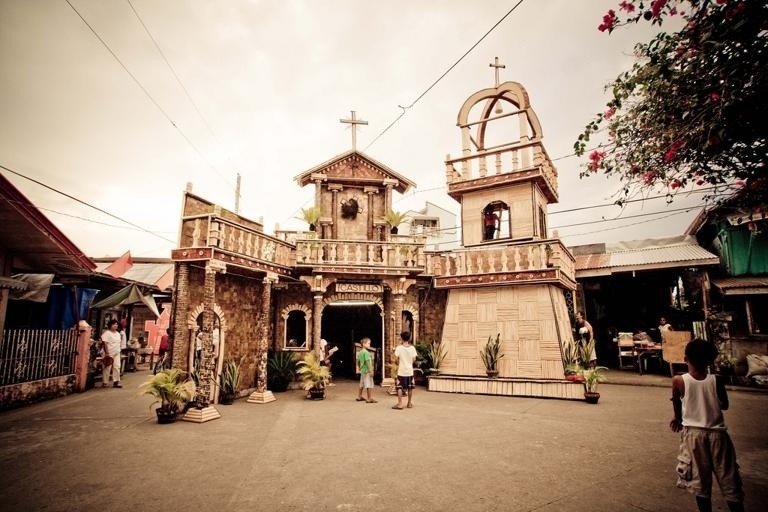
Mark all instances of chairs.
[91,345,155,376]
[618,332,636,369]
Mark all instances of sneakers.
[113,381,123,388]
[101,382,109,388]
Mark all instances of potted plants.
[269,349,298,391]
[479,333,504,378]
[386,338,448,395]
[134,368,201,424]
[295,347,331,399]
[562,337,610,403]
[292,206,328,230]
[384,206,419,234]
[211,354,247,405]
[399,246,419,267]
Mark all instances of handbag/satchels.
[103,357,114,366]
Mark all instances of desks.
[636,341,662,374]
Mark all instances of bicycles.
[154,356,169,376]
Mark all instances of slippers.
[392,404,404,409]
[407,402,413,408]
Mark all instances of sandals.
[356,397,367,401]
[366,399,378,403]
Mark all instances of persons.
[391,331,417,409]
[658,317,673,370]
[633,330,652,364]
[320,337,334,384]
[485,208,501,240]
[608,327,628,370]
[89,313,144,387]
[576,311,598,369]
[669,339,745,512]
[356,336,379,403]
[153,328,173,375]
